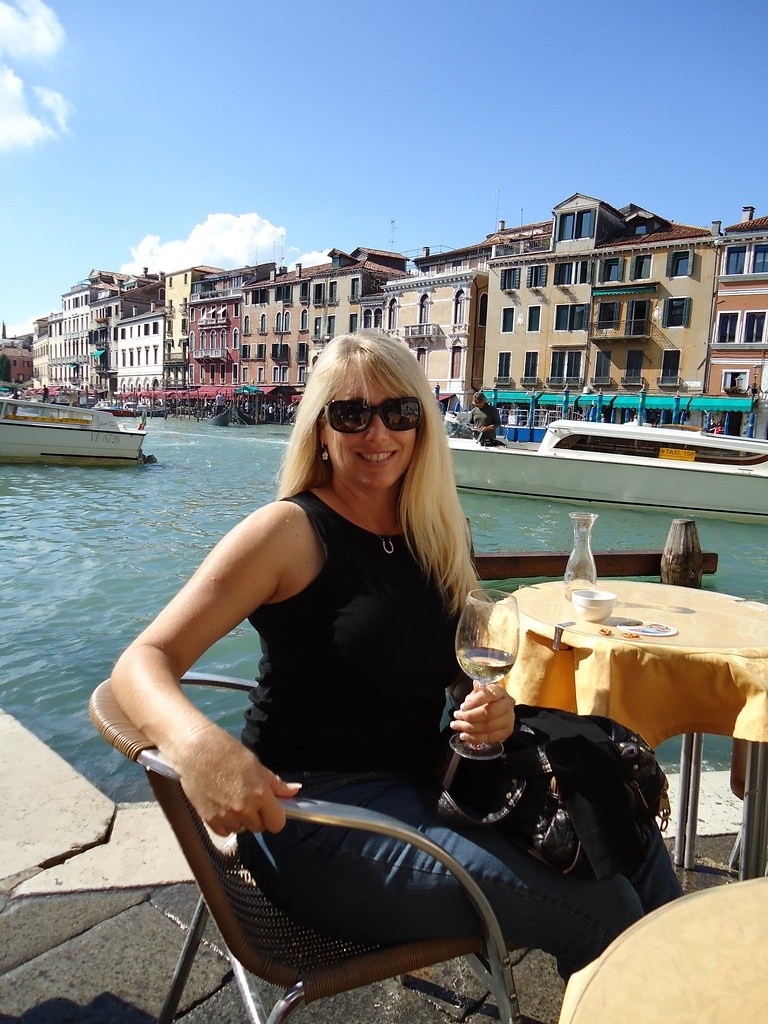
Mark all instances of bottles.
[564,512,599,601]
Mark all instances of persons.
[244,401,294,423]
[110,331,686,985]
[633,415,638,426]
[709,418,724,435]
[42,385,49,403]
[204,393,211,418]
[216,392,224,416]
[600,414,605,423]
[467,391,501,446]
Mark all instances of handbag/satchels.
[481,438,507,448]
[437,701,670,880]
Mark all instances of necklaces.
[333,485,399,554]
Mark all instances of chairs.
[87,672,529,1024]
[6,414,92,425]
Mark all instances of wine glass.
[449,589,519,760]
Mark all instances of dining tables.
[558,874,768,1024]
[480,579,767,882]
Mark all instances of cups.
[571,591,617,622]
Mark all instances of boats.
[208,409,231,427]
[0,398,147,466]
[446,420,768,524]
[91,399,170,417]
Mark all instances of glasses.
[323,397,422,434]
[476,402,482,404]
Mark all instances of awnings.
[69,364,79,369]
[93,349,105,358]
[481,389,756,412]
[116,390,195,399]
[86,389,108,396]
[283,395,302,403]
[192,386,276,401]
[434,393,454,402]
[37,385,63,395]
